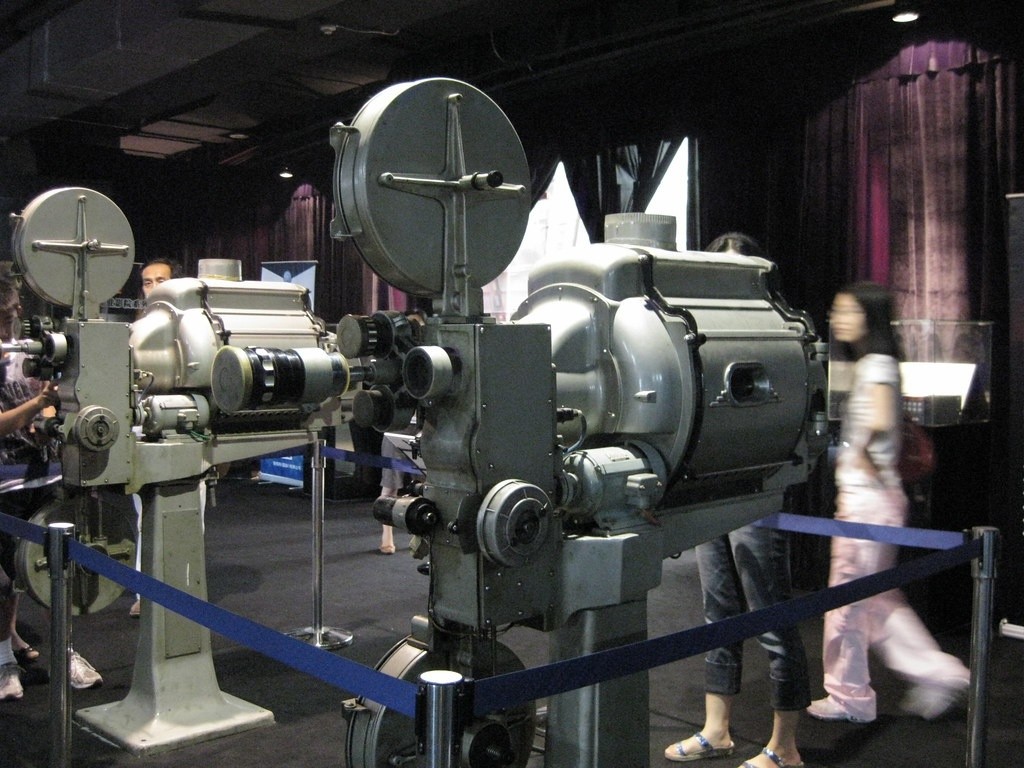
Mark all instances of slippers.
[665,732,734,761]
[740,746,804,768]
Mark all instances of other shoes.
[130,600,141,616]
[380,544,395,554]
[13,645,40,660]
[924,685,966,719]
[806,696,875,722]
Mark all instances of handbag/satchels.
[900,415,933,483]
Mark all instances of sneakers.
[0,662,27,699]
[48,649,103,688]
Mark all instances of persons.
[129,257,207,618]
[806,285,971,725]
[0,261,103,713]
[664,231,804,768]
[379,308,428,555]
